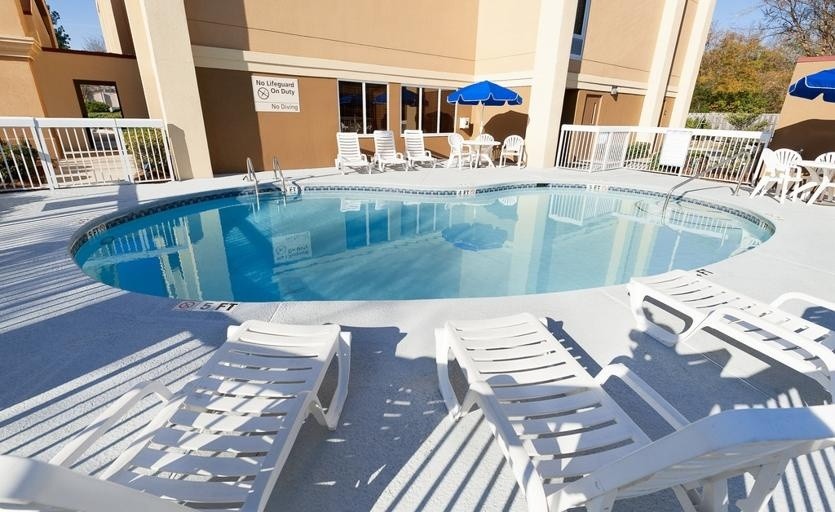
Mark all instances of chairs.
[749,148,835,207]
[335,129,525,174]
[434,310,835,512]
[1,320,352,512]
[627,269,835,405]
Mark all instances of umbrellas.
[788,68,835,103]
[372,87,426,105]
[448,81,522,164]
[341,92,371,105]
[440,207,509,252]
